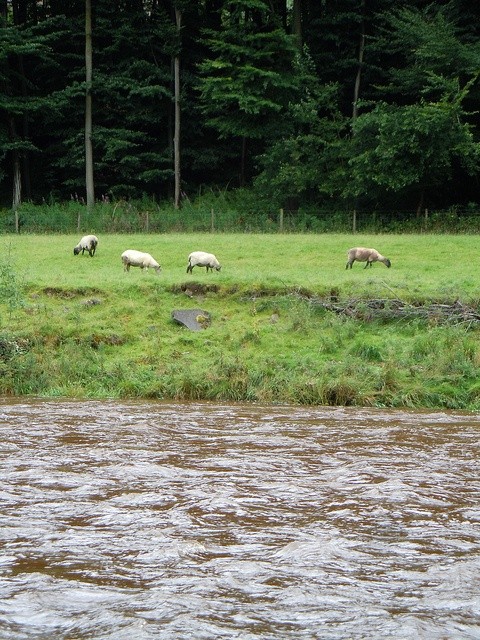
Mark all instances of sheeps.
[344,248,391,270]
[121,250,161,275]
[186,251,222,274]
[74,235,98,257]
